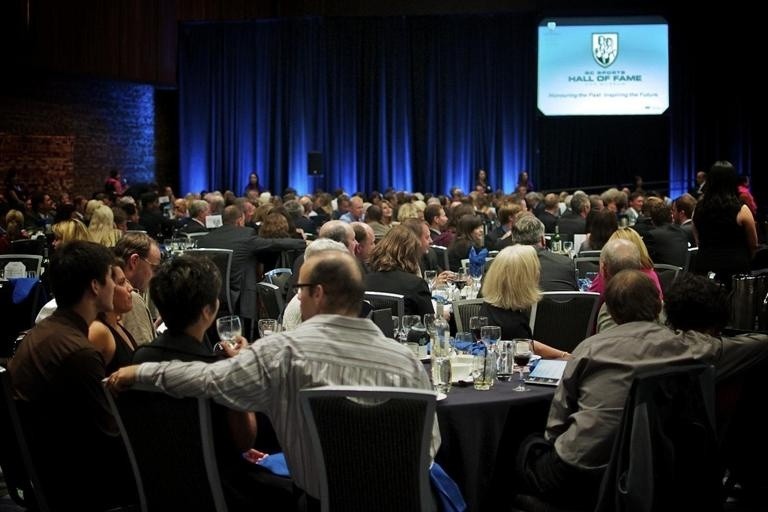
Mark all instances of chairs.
[532,362,721,506]
[396,350,562,507]
[101,373,232,508]
[297,386,447,509]
[1,196,768,347]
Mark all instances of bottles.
[40,246,50,279]
[550,225,561,253]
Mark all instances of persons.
[1,162,767,512]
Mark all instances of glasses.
[292,282,312,295]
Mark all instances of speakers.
[307,152,324,178]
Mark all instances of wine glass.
[425,268,477,302]
[563,242,573,253]
[215,316,243,348]
[512,338,535,392]
[163,236,195,252]
[390,312,512,401]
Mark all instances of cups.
[257,318,281,337]
[574,268,598,292]
